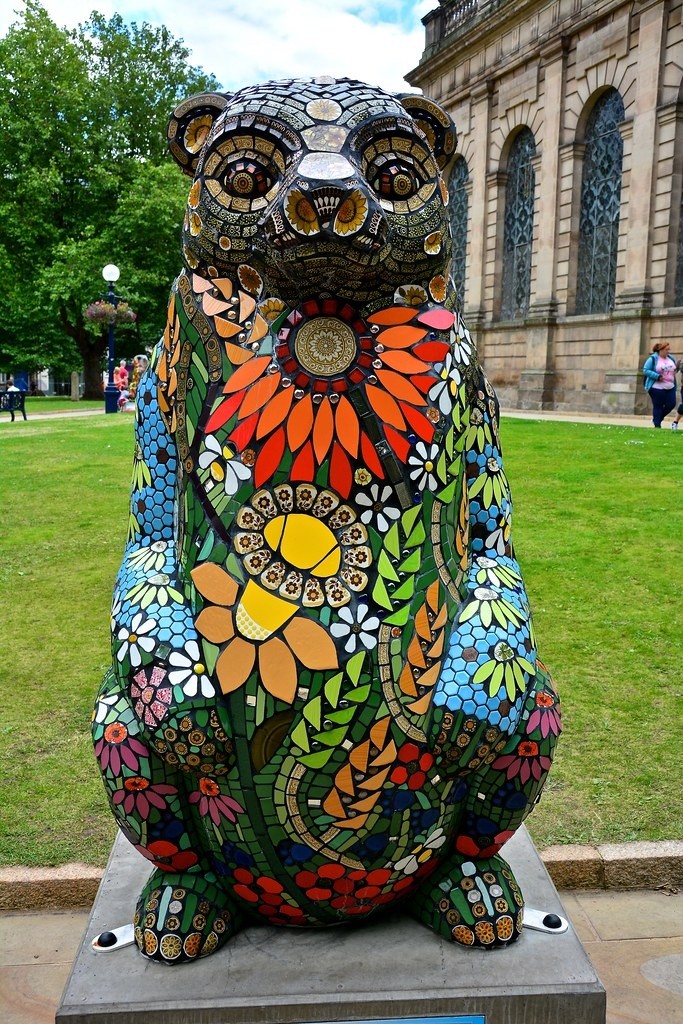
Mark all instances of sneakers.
[672,423,677,430]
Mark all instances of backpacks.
[641,352,676,386]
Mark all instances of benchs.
[0,391,27,422]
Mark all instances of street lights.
[82,263,137,414]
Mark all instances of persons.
[642,340,682,429]
[3,379,28,422]
[30,381,38,396]
[670,354,683,431]
[112,358,130,411]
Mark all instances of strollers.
[118,385,137,412]
